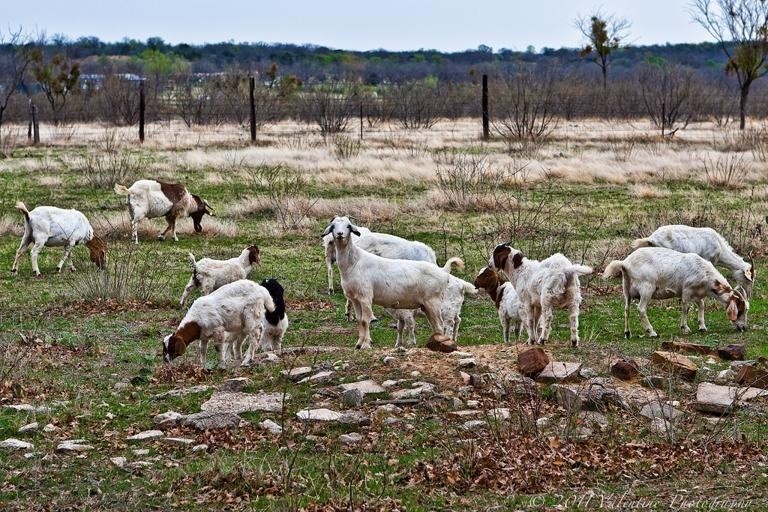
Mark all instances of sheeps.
[487,240,593,349]
[161,278,288,368]
[179,243,262,307]
[629,223,756,299]
[321,213,479,351]
[601,245,750,340]
[473,265,542,344]
[114,178,217,245]
[9,199,110,279]
